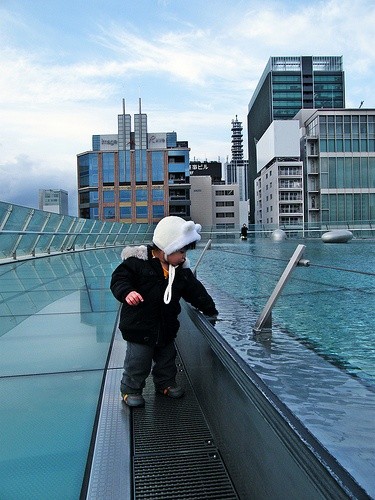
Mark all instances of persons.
[110,216,219,406]
[241,223,248,240]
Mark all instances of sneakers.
[121,390,146,406]
[154,383,183,398]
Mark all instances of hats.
[152,216,202,254]
